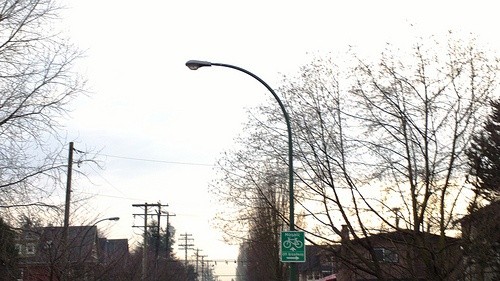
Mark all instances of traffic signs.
[280,230,306,263]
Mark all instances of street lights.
[186,60,298,281]
[80,217,119,265]
